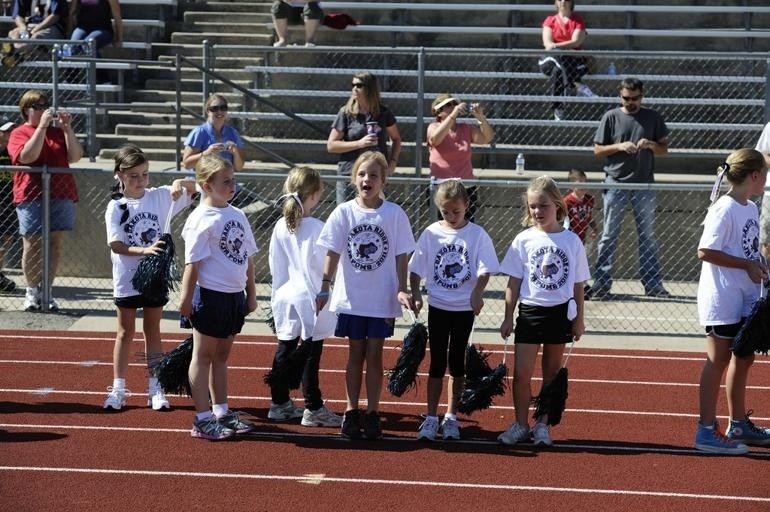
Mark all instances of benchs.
[0,0,180,140]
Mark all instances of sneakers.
[190,417,235,439]
[589,288,610,301]
[532,422,553,446]
[365,412,384,440]
[341,410,363,440]
[220,410,256,433]
[553,108,568,119]
[268,400,304,419]
[103,384,132,410]
[26,289,56,312]
[497,422,529,445]
[726,418,770,441]
[645,288,671,298]
[146,386,171,411]
[695,420,750,454]
[416,415,439,441]
[302,406,342,427]
[442,414,460,441]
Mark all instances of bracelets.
[323,279,331,282]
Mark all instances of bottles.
[608,62,617,75]
[53,39,93,58]
[516,151,526,176]
[21,31,30,40]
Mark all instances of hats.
[432,94,461,111]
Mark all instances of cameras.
[465,103,473,113]
[51,113,59,120]
[221,143,229,151]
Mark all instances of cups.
[365,121,379,145]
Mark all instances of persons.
[266,167,342,426]
[102,144,196,412]
[562,167,597,300]
[270,0,325,47]
[587,79,672,301]
[693,147,770,455]
[499,176,592,448]
[180,156,259,438]
[326,73,400,204]
[426,93,493,221]
[755,121,770,261]
[538,0,599,120]
[183,96,244,209]
[0,0,124,309]
[315,152,417,441]
[408,181,498,440]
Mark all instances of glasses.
[620,94,641,102]
[25,101,52,111]
[208,104,229,112]
[352,82,363,88]
